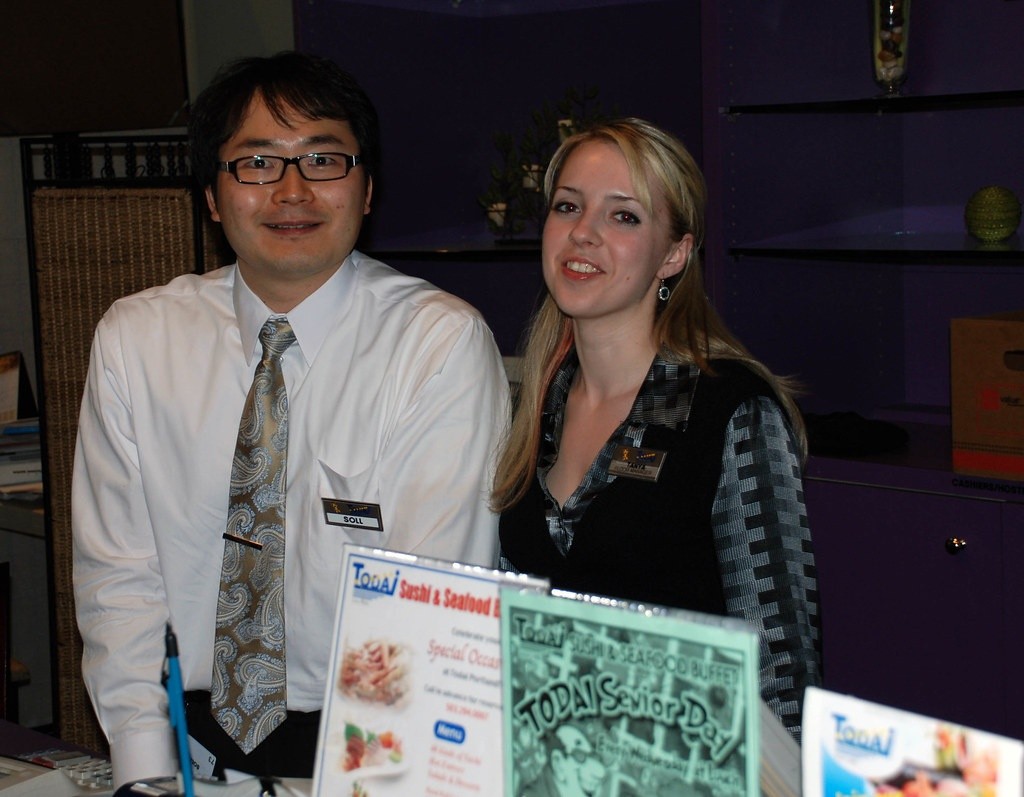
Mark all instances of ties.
[210,320,297,755]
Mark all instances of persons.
[489,116,822,748]
[72,52,512,797]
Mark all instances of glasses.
[219,151,362,185]
